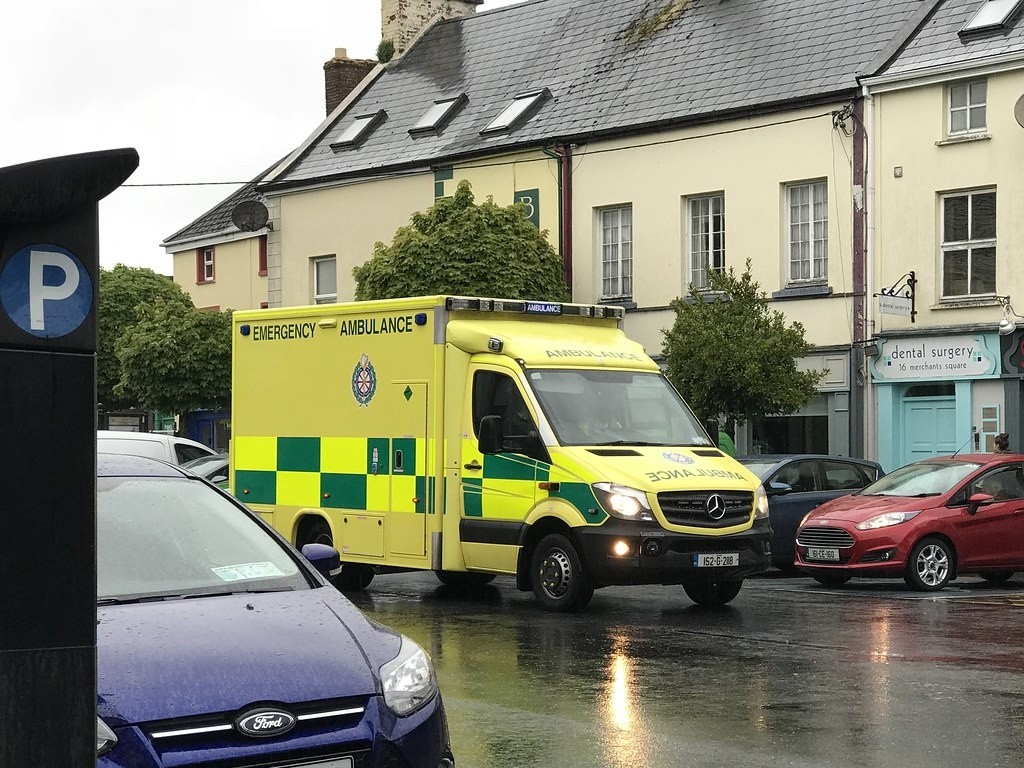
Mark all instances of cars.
[96,451,455,768]
[97,429,219,465]
[177,453,229,483]
[794,454,1024,592]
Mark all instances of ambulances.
[229,294,773,615]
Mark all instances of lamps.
[999,304,1024,336]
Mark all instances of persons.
[504,395,542,453]
[993,433,1015,454]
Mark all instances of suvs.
[730,455,885,573]
